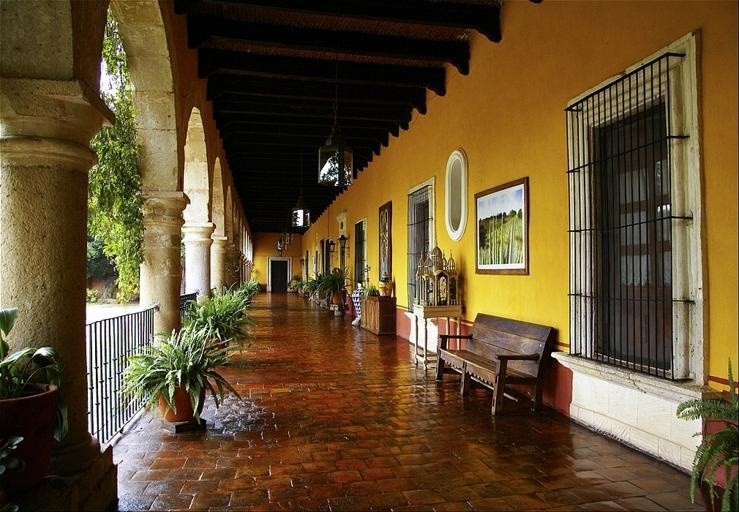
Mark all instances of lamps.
[328,240,337,259]
[337,235,350,257]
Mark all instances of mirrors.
[443,148,468,242]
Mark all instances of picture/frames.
[379,201,392,281]
[473,176,529,275]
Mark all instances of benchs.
[435,313,556,417]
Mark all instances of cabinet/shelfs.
[360,295,396,335]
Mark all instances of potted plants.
[368,284,379,295]
[291,265,352,304]
[0,435,24,509]
[0,306,65,489]
[118,319,244,422]
[184,278,260,366]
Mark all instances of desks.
[412,304,463,378]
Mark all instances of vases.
[351,283,368,316]
